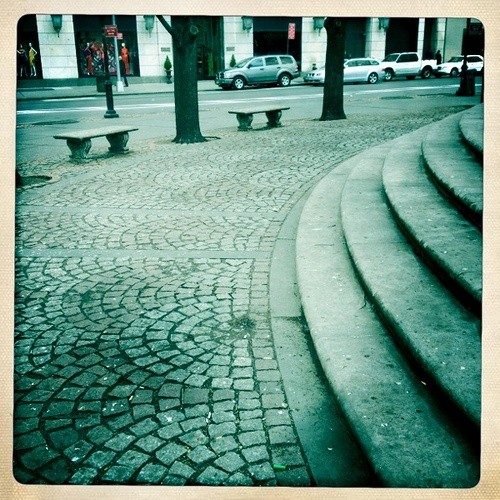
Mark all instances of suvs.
[215,54,299,90]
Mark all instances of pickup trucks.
[380,52,438,82]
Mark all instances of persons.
[435,50,442,65]
[84,42,95,75]
[117,55,128,87]
[17,44,28,78]
[119,43,131,75]
[98,43,107,71]
[27,42,38,77]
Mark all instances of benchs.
[226,105,291,130]
[54,126,139,160]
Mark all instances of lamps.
[143,15,155,37]
[241,16,254,36]
[379,17,389,35]
[51,14,63,38]
[312,17,326,37]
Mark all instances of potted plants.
[164,55,173,84]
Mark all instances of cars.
[303,57,382,86]
[435,54,484,78]
